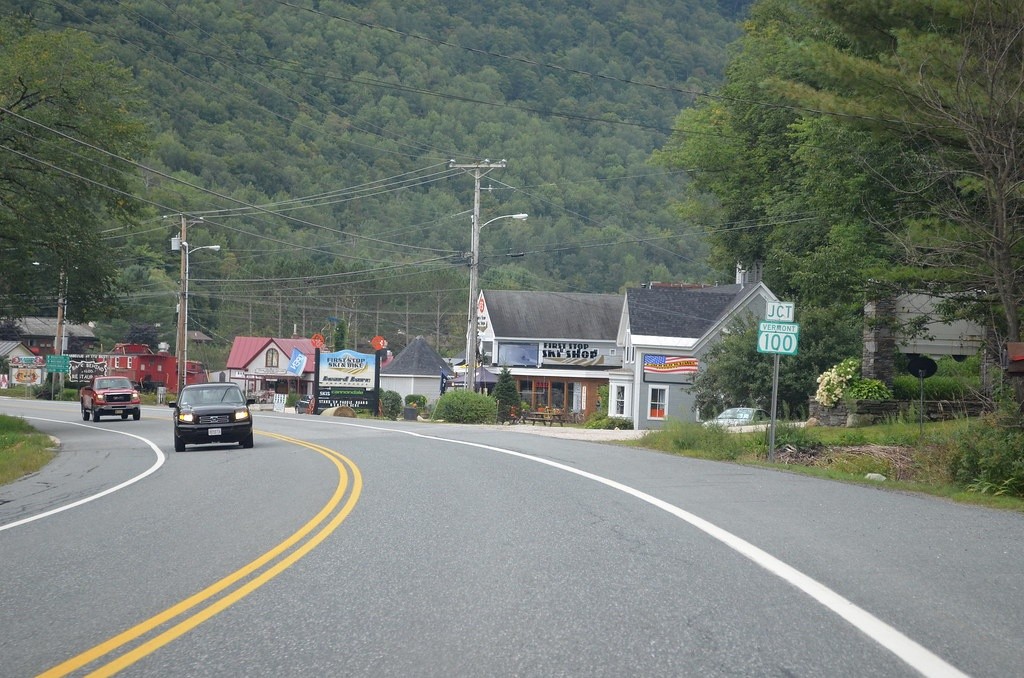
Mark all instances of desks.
[549,414,563,427]
[530,411,551,425]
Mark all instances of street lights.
[180,242,221,367]
[471,210,527,392]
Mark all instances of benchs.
[525,417,566,423]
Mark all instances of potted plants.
[419,408,429,419]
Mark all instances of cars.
[78,372,143,423]
[167,382,255,453]
[701,407,781,430]
